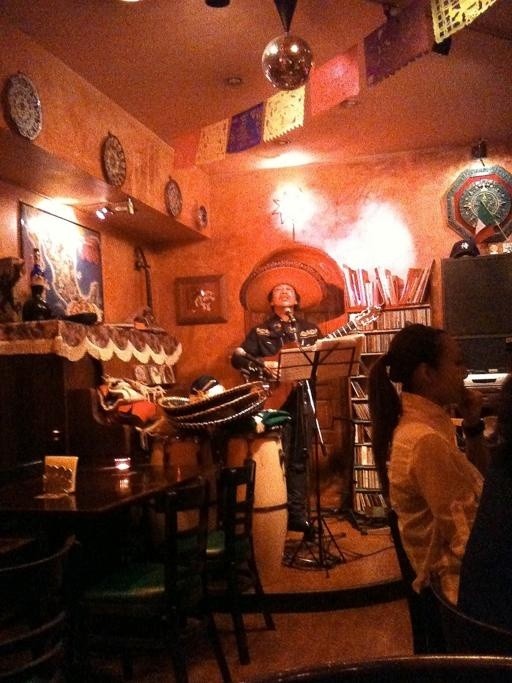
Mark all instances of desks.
[0,461,207,518]
[1,317,183,468]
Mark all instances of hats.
[157,376,267,429]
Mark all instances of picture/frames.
[175,274,227,326]
[16,199,105,325]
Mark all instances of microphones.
[283,304,298,330]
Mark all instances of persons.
[75,235,103,304]
[455,368,511,629]
[367,319,491,608]
[230,283,326,537]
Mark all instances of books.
[278,333,367,382]
[343,263,431,512]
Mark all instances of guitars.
[136,245,157,328]
[246,304,382,410]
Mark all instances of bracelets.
[461,419,485,438]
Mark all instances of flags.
[473,197,497,245]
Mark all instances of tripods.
[286,425,348,578]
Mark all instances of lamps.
[270,185,313,243]
[94,198,135,220]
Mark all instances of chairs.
[252,656,511,683]
[386,510,511,657]
[0,461,275,682]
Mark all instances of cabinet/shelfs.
[345,304,432,520]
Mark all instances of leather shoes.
[288,518,324,534]
